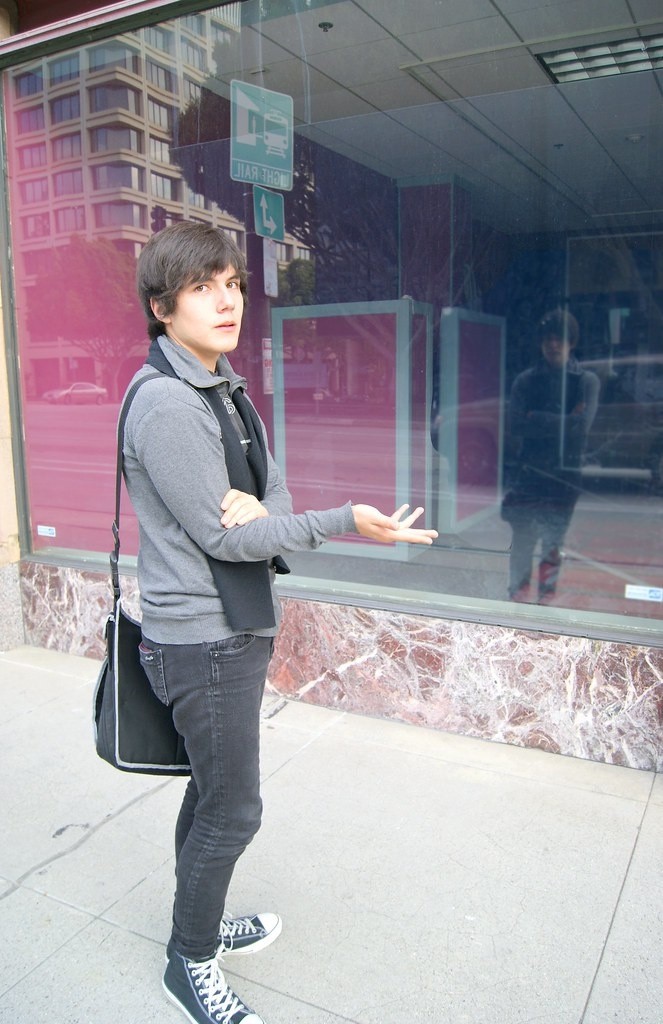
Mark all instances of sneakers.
[165,912,282,966]
[161,952,265,1024]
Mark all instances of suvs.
[430,353,663,487]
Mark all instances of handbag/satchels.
[94,599,193,776]
[501,488,518,524]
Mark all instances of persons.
[496,309,600,605]
[117,221,439,1024]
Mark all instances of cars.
[41,381,109,406]
